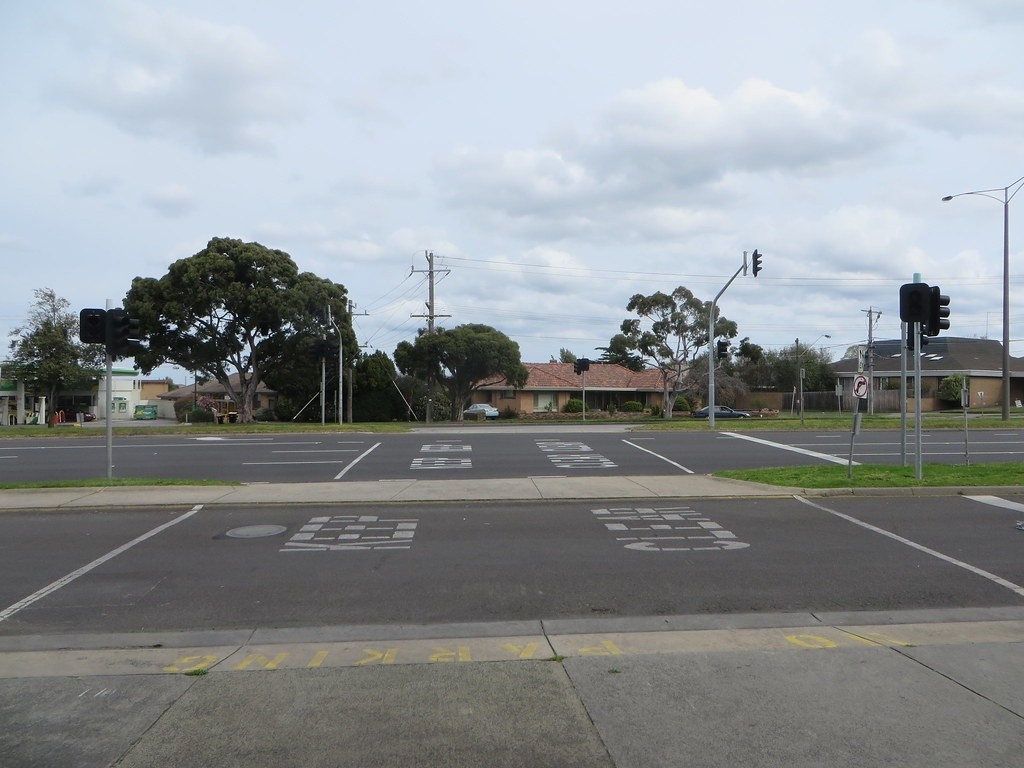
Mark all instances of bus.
[133,405,158,420]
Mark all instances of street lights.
[796,333,831,415]
[941,176,1024,421]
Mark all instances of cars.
[691,405,750,419]
[462,403,499,420]
[56,407,96,423]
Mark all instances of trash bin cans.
[228,412,237,423]
[217,415,225,424]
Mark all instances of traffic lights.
[899,283,930,323]
[573,359,581,375]
[753,248,763,276]
[581,358,589,371]
[105,309,130,345]
[907,322,930,351]
[128,318,141,340]
[79,308,106,343]
[929,286,950,337]
